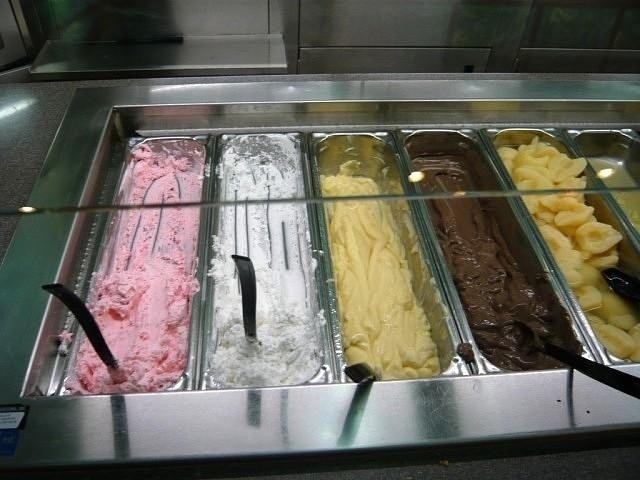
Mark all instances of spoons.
[601,266,640,301]
[512,318,640,403]
